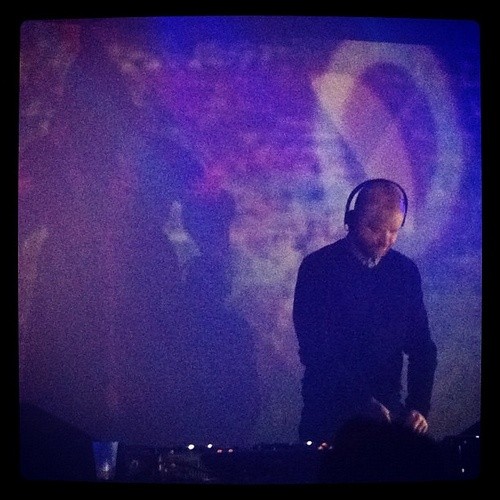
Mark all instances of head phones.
[343,178,408,229]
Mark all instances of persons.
[294,180,442,446]
